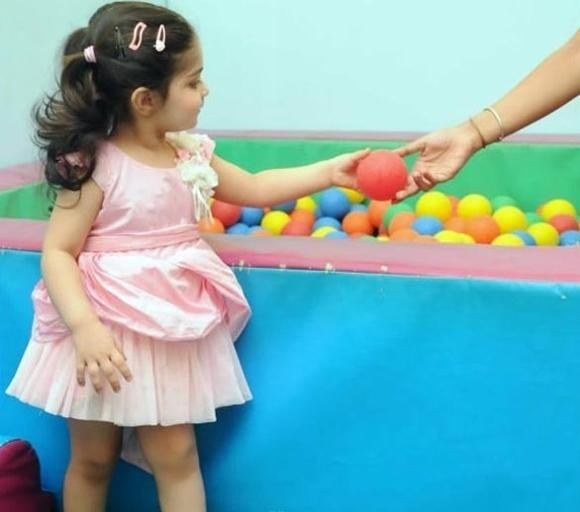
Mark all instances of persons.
[4,1,372,512]
[391,27,580,202]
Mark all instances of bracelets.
[483,107,505,142]
[468,118,486,147]
